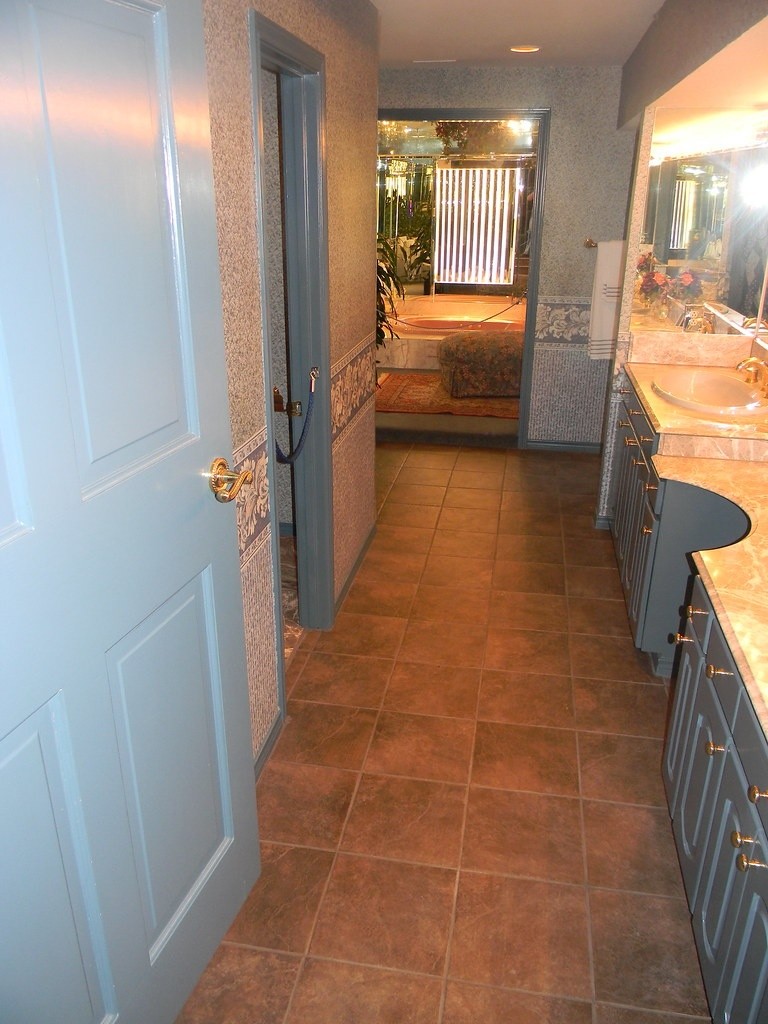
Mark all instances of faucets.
[686,317,715,334]
[742,317,768,331]
[735,357,768,392]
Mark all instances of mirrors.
[631,10,766,360]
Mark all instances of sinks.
[651,369,768,416]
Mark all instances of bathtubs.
[376,314,525,370]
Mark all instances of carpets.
[376,370,521,420]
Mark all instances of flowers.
[635,252,703,306]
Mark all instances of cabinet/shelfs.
[601,362,768,1023]
